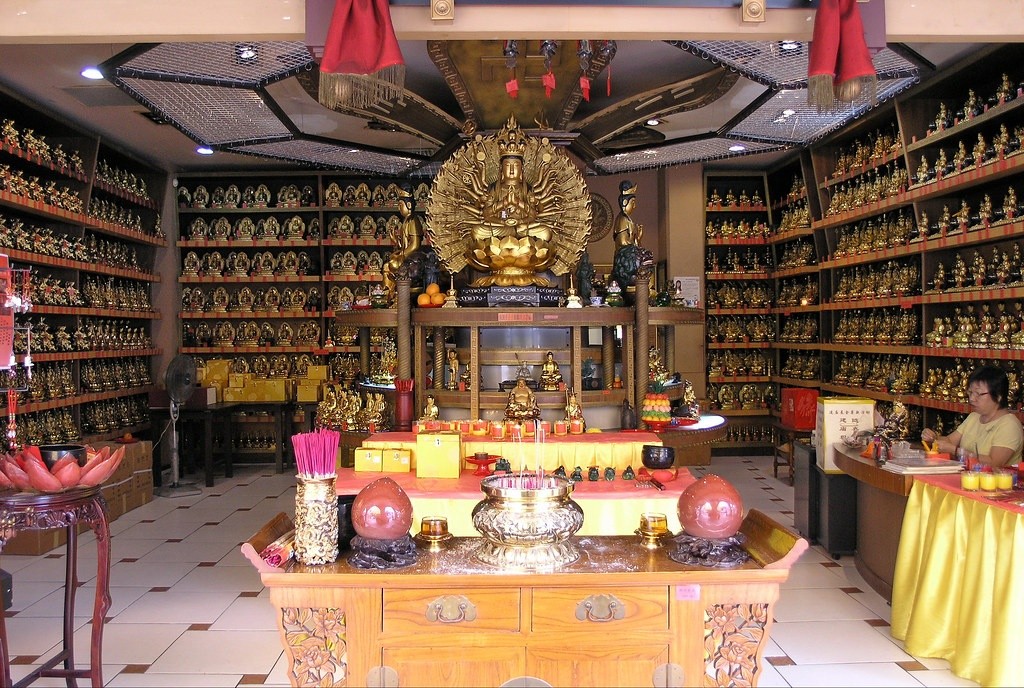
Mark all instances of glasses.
[967,389,991,397]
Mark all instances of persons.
[920,364,1024,474]
[683,378,697,405]
[565,393,583,421]
[648,345,671,380]
[0,114,452,453]
[451,140,568,243]
[503,375,541,413]
[540,351,563,385]
[447,347,459,382]
[704,67,1024,446]
[515,359,531,379]
[613,179,656,277]
[674,280,683,294]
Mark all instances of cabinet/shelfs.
[703,42,1023,454]
[0,87,433,465]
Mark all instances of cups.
[996,470,1013,492]
[979,470,997,492]
[960,471,979,492]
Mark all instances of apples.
[646,393,668,400]
[613,376,621,387]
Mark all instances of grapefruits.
[417,282,447,305]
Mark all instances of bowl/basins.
[641,445,675,469]
[37,444,88,470]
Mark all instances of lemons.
[641,416,671,421]
[643,399,670,406]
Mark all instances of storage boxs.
[816,396,876,475]
[781,387,819,431]
[0,360,462,557]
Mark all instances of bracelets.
[954,445,960,458]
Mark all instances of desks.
[792,440,856,560]
[147,400,319,488]
[0,485,112,688]
[770,411,810,486]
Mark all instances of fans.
[154,354,201,498]
